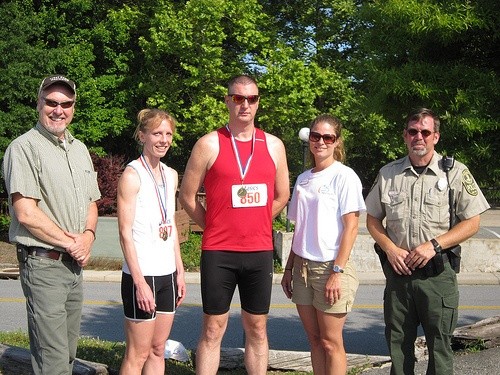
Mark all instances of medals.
[237,188,247,198]
[162,231,168,241]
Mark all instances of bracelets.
[83,228,96,241]
[285,268,292,271]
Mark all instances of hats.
[38,75,75,97]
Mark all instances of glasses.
[407,128,435,136]
[228,94,259,104]
[42,96,74,108]
[309,129,336,144]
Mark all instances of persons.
[365,107,490,375]
[281,113,368,375]
[3,75,101,375]
[178,76,290,375]
[117,108,187,375]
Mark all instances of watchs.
[331,265,344,274]
[430,238,442,255]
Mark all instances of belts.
[27,246,73,262]
